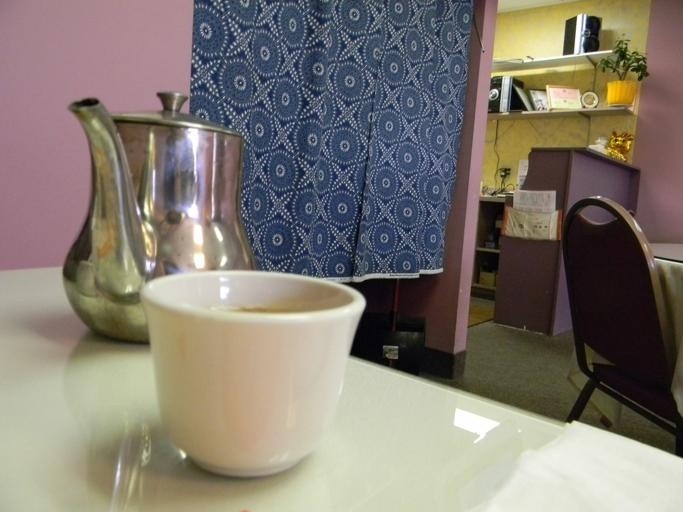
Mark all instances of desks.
[0,265,683,512]
[561,242,683,432]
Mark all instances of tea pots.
[59,91,257,341]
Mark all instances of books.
[511,188,558,216]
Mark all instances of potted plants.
[596,32,649,107]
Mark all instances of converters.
[500,168,506,177]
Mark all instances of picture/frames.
[512,84,583,112]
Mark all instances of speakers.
[488,75,523,112]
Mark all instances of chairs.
[562,191,683,459]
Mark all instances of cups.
[141,272,368,477]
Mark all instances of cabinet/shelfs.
[487,49,634,119]
[469,196,505,301]
